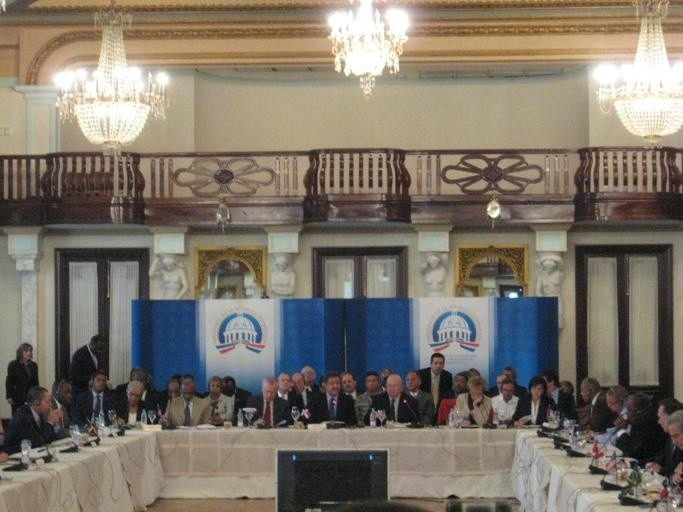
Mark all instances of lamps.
[591,0,683,148]
[51,0,171,163]
[325,0,412,104]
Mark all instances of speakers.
[277,449,387,511]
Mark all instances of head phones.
[31,398,42,407]
[586,387,593,396]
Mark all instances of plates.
[196,424,214,429]
[306,423,327,429]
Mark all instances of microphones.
[7,396,53,462]
[589,427,620,474]
[401,399,424,428]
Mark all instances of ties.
[432,375,439,412]
[184,401,190,425]
[327,396,337,420]
[94,394,101,421]
[263,400,271,425]
[390,398,395,421]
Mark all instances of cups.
[563,419,576,434]
[94,416,104,441]
[223,421,232,428]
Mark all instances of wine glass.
[666,486,681,512]
[67,425,79,446]
[291,407,300,422]
[108,410,117,427]
[447,409,559,429]
[19,439,30,462]
[148,411,156,423]
[245,411,253,428]
[377,409,385,430]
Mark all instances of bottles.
[237,408,243,426]
[369,407,375,429]
[140,409,147,423]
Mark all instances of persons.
[267,256,298,298]
[533,256,564,332]
[0,351,683,510]
[3,341,38,419]
[419,252,448,297]
[68,334,104,400]
[147,254,189,299]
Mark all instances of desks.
[517,425,683,511]
[135,424,538,512]
[1,424,155,512]
